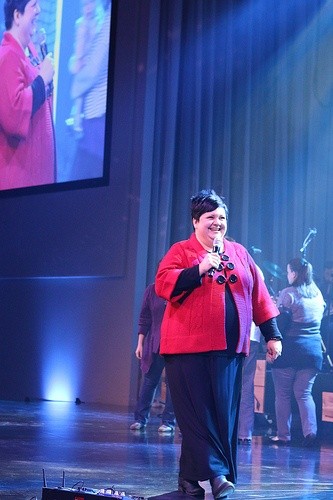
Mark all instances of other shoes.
[304,433,318,448]
[268,436,290,446]
[209,474,235,499]
[238,436,253,446]
[178,477,206,497]
[129,423,145,431]
[157,425,175,432]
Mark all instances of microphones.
[208,235,222,277]
[38,28,55,90]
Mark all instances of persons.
[154,191,283,500]
[65,0,112,182]
[129,256,333,448]
[0,0,58,191]
